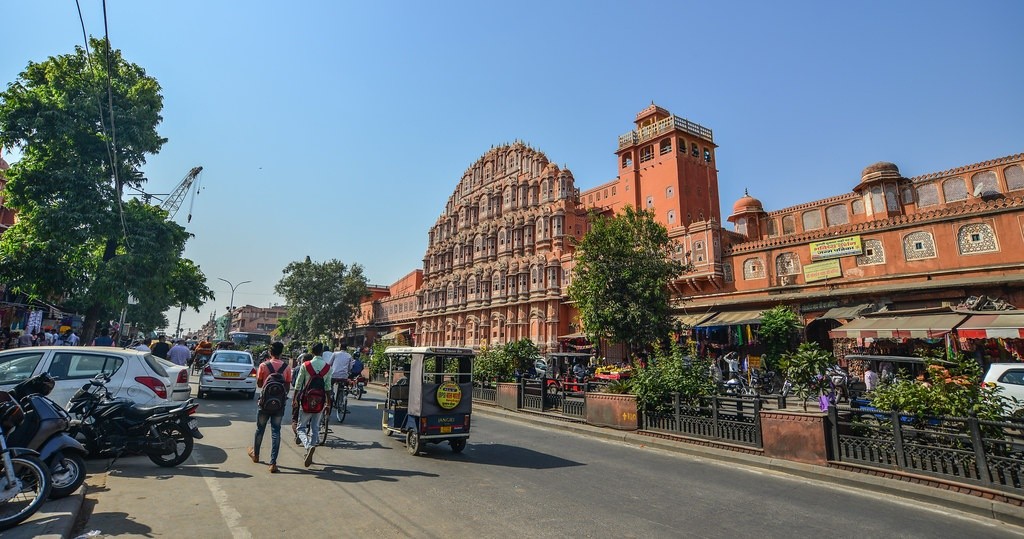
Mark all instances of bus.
[228,332,271,348]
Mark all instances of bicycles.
[330,381,351,424]
[305,403,334,446]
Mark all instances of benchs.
[388,384,409,412]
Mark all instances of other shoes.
[268,464,276,472]
[248,447,258,462]
[296,437,302,444]
[304,446,315,466]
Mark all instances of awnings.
[829,314,1024,340]
[667,304,790,327]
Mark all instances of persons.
[246,341,364,475]
[573,361,587,390]
[849,361,894,392]
[710,350,767,394]
[0,323,198,371]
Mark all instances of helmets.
[353,351,360,359]
[732,352,738,359]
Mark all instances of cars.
[197,350,257,400]
[0,345,173,459]
[981,362,1024,435]
[0,354,191,401]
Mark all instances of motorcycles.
[548,353,602,396]
[0,389,52,531]
[723,368,757,401]
[348,376,368,400]
[63,371,204,468]
[830,370,859,405]
[376,346,475,455]
[749,368,776,395]
[0,371,88,498]
[779,378,795,397]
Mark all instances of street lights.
[218,277,252,340]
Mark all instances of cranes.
[160,166,203,223]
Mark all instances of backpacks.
[257,360,288,412]
[299,361,330,413]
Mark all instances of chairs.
[50,362,66,378]
[238,359,247,362]
[216,358,226,362]
